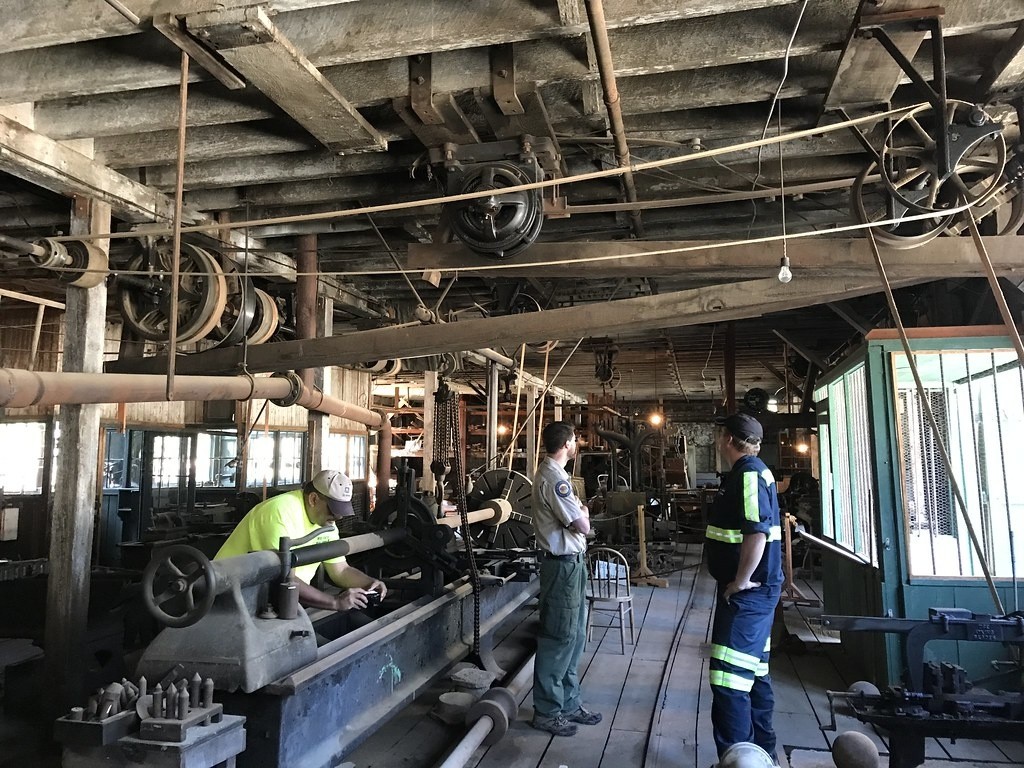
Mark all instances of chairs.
[584,547,635,655]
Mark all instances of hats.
[715,413,763,445]
[312,470,355,516]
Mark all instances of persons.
[206,469,388,612]
[704,413,786,768]
[529,420,603,738]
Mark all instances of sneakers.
[530,704,579,736]
[561,704,602,725]
